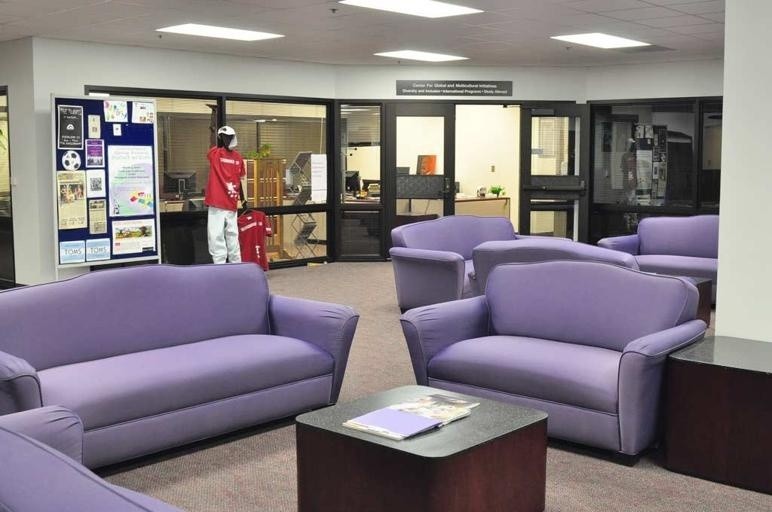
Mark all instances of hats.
[218,126,235,135]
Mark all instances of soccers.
[62,151,81,170]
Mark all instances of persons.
[621,124,640,231]
[204,104,247,264]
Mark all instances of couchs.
[0,262,360,468]
[467,239,637,294]
[400,260,706,457]
[389,214,576,312]
[598,213,719,304]
[0,404,189,512]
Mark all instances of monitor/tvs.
[361,179,381,189]
[396,166,409,174]
[345,170,359,193]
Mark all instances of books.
[345,394,480,441]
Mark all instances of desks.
[161,201,213,264]
[295,384,547,511]
[662,336,771,494]
[668,273,711,329]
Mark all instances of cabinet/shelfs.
[239,158,286,257]
